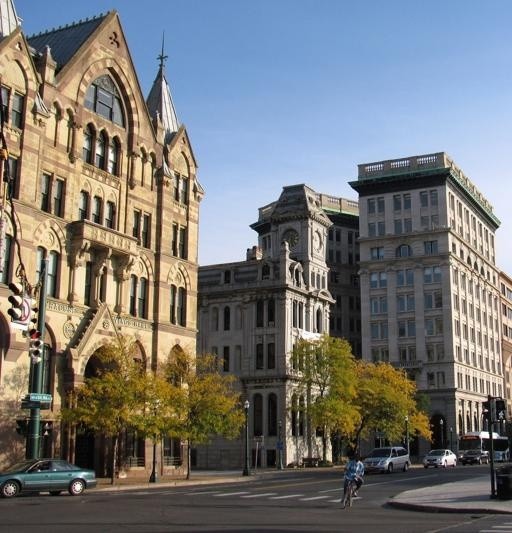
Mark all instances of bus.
[456,429,510,463]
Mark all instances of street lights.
[439,418,445,449]
[147,397,161,483]
[241,398,250,475]
[276,418,285,470]
[404,415,410,452]
[450,425,454,451]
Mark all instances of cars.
[458,449,489,466]
[421,448,458,469]
[1,456,99,498]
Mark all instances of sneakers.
[351,490,358,497]
[343,497,348,502]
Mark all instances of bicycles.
[343,475,359,512]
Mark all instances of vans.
[361,445,412,474]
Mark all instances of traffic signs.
[29,392,53,402]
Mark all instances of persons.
[339,454,365,504]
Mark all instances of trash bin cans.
[496,464,512,499]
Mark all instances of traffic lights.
[6,280,24,323]
[30,337,43,363]
[21,297,39,336]
[40,420,52,437]
[15,418,29,435]
[495,399,507,420]
[481,400,490,419]
